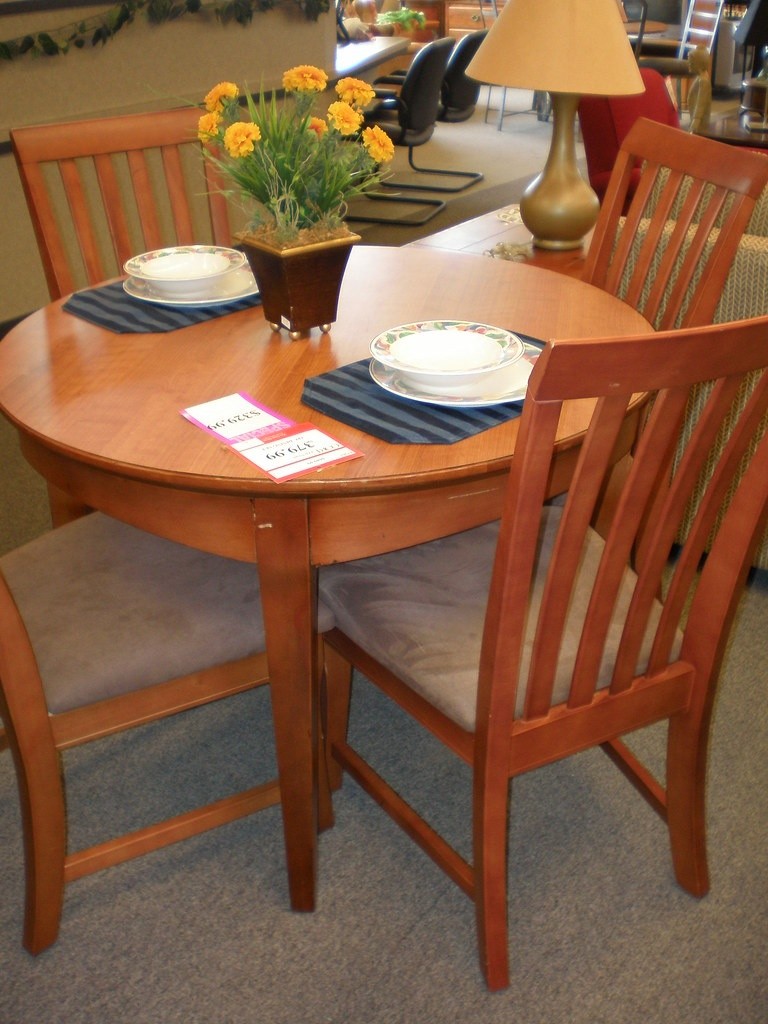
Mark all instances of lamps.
[730,0,768,80]
[461,0,646,252]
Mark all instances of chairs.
[371,26,491,194]
[638,0,724,118]
[0,513,339,959]
[478,0,549,131]
[12,104,241,533]
[548,119,768,606]
[315,313,768,996]
[339,36,457,227]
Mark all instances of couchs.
[606,161,768,571]
[577,69,768,218]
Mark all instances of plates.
[369,342,542,407]
[122,260,259,309]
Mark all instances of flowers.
[375,7,426,32]
[184,64,398,242]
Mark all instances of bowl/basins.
[369,319,525,396]
[122,244,246,299]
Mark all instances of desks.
[0,244,657,914]
[337,37,407,85]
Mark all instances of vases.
[230,233,361,338]
[394,29,416,42]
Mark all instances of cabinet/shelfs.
[445,0,508,44]
